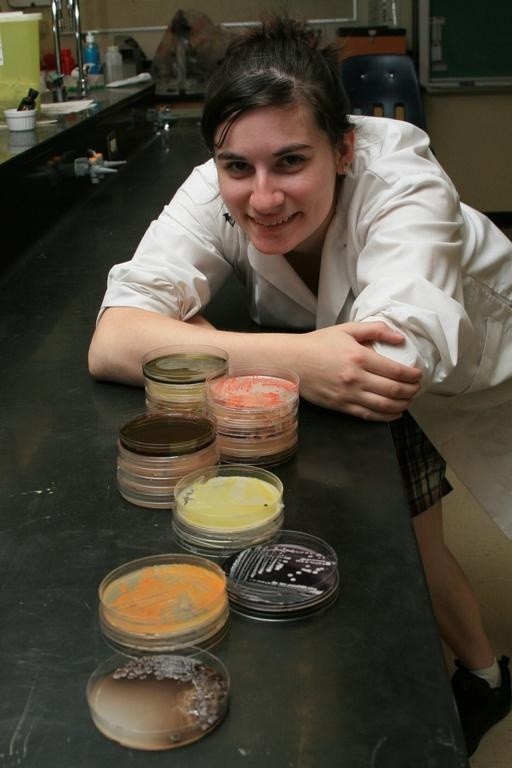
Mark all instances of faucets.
[68,0,83,79]
[51,0,65,75]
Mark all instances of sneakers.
[451,656,510,759]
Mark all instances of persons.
[88,13,512,759]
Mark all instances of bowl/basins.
[3,108,37,131]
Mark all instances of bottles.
[16,88,39,110]
[104,47,123,85]
